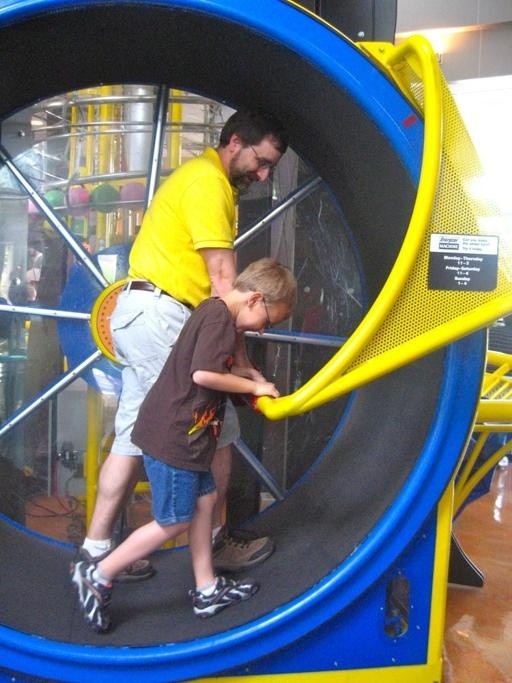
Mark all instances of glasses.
[248,142,270,169]
[262,298,274,329]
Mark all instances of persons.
[69,256,299,632]
[71,108,288,584]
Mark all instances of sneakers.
[188,576,258,619]
[211,524,274,576]
[69,546,153,582]
[71,561,114,635]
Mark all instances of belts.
[123,282,192,308]
